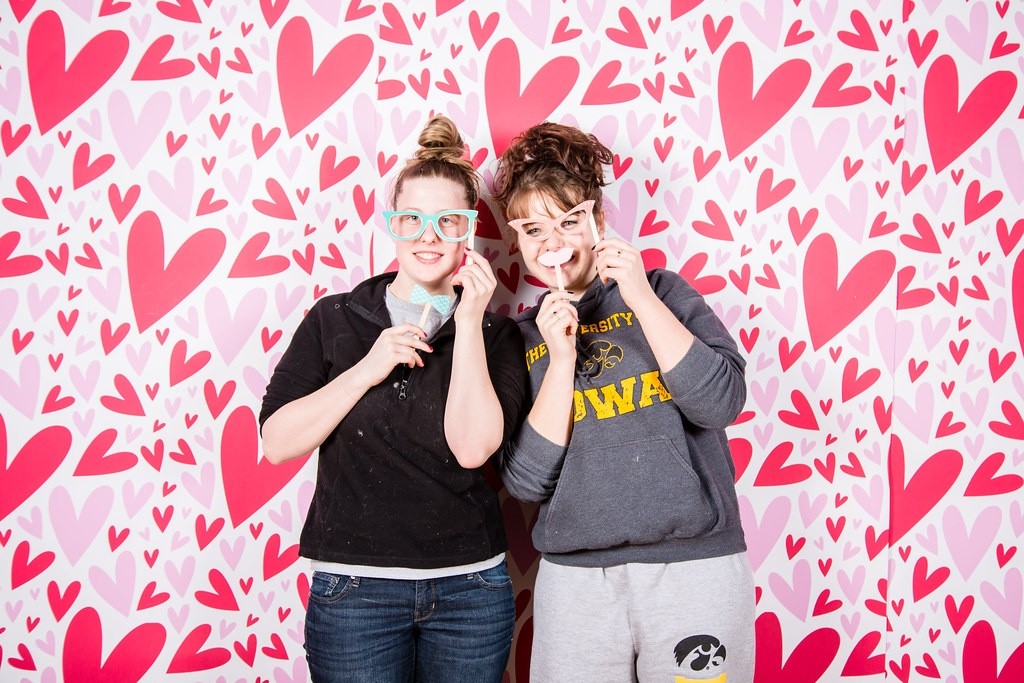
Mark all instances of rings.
[617,249,623,257]
[553,310,561,320]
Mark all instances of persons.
[258,115,531,683]
[496,123,756,683]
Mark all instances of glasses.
[382,210,478,242]
[507,199,595,241]
